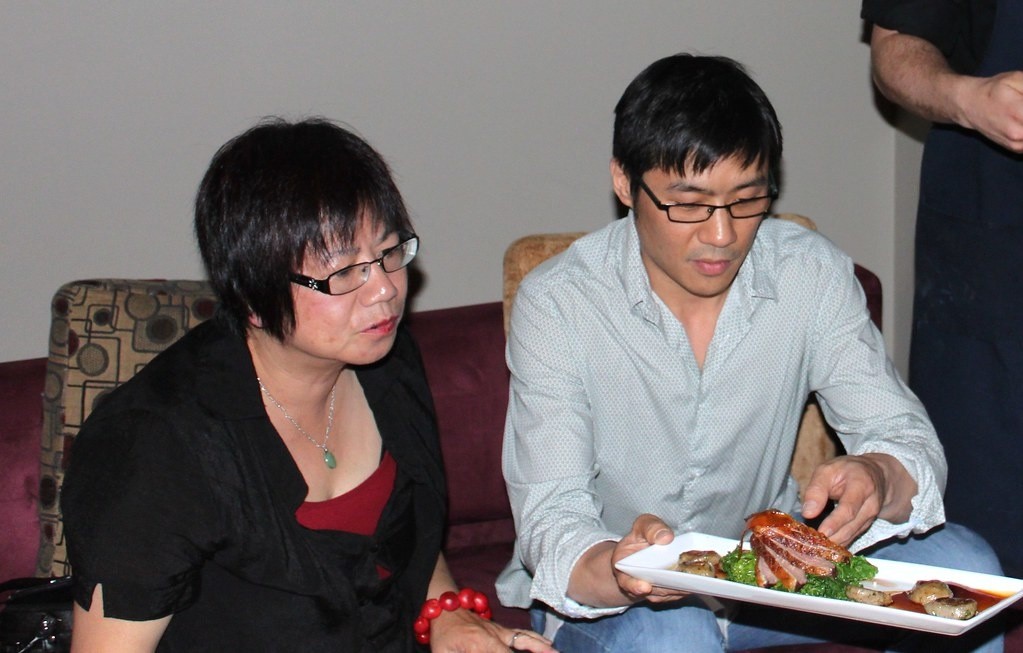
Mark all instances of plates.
[614,532,1023,636]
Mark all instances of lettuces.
[721,545,879,601]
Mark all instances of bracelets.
[412,588,494,646]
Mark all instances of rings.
[511,631,522,648]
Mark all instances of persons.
[489,51,1009,653]
[60,117,556,653]
[860,0,1023,628]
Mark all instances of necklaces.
[254,376,338,468]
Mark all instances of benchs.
[0,262,882,639]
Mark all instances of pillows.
[33,280,235,581]
[503,217,836,501]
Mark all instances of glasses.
[280,230,420,295]
[632,169,778,223]
